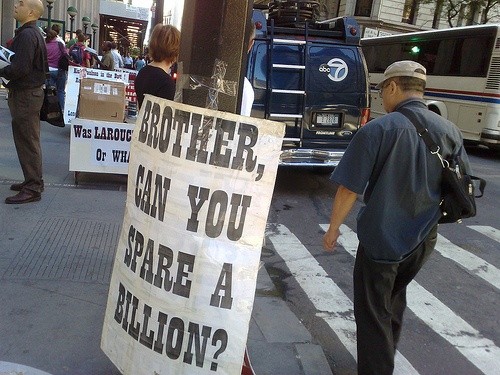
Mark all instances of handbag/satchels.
[38,83,66,128]
[434,155,476,223]
[58,53,72,70]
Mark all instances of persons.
[321,60,481,373]
[42,24,73,128]
[241,20,258,118]
[66,29,146,71]
[0,0,47,205]
[7,27,19,46]
[134,24,181,110]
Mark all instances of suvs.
[246,14,372,168]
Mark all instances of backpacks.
[70,45,83,63]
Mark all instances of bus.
[352,24,499,150]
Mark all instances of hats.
[374,60,427,88]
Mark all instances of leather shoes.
[10,180,44,191]
[6,188,41,204]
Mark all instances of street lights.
[91,23,99,51]
[46,0,53,28]
[66,6,79,40]
[81,16,90,41]
